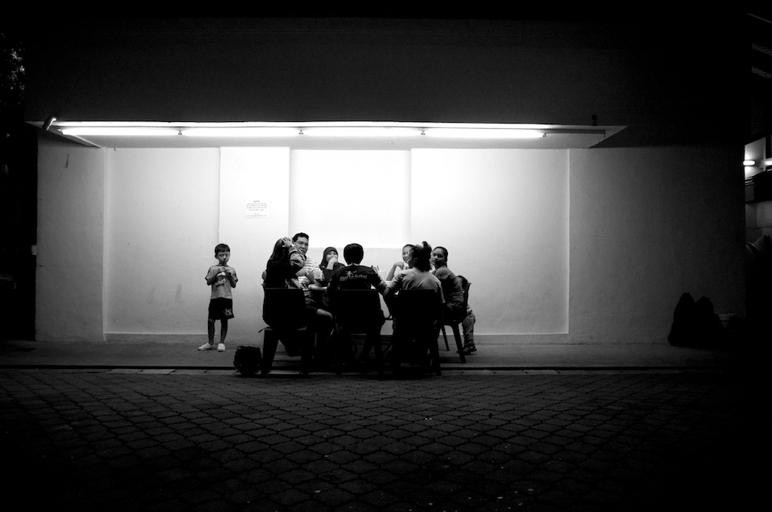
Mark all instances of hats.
[324,247,338,258]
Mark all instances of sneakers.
[217,342,225,352]
[198,341,217,350]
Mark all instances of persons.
[197,244,238,352]
[261,233,477,379]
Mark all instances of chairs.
[255,271,472,381]
